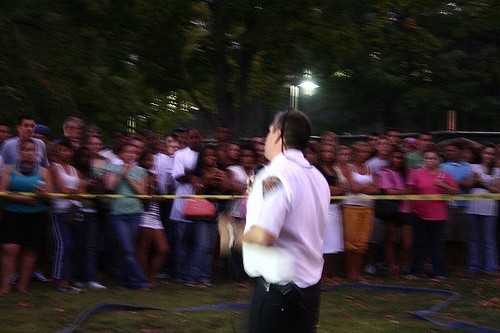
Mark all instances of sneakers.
[35,272,47,281]
[58,284,88,294]
[88,281,106,289]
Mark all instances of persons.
[216,106,330,333]
[0,115,500,298]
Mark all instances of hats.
[34,125,49,136]
[400,138,418,149]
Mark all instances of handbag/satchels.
[65,205,84,227]
[374,190,400,219]
[182,185,217,220]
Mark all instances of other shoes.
[96,271,111,278]
[10,273,20,284]
[238,285,249,291]
[423,263,433,271]
[464,272,476,279]
[186,282,214,289]
[322,276,343,285]
[1,286,11,294]
[431,277,447,285]
[353,278,372,285]
[138,280,161,290]
[391,274,420,280]
[116,286,129,290]
[16,287,32,295]
[484,271,500,278]
[364,265,376,274]
